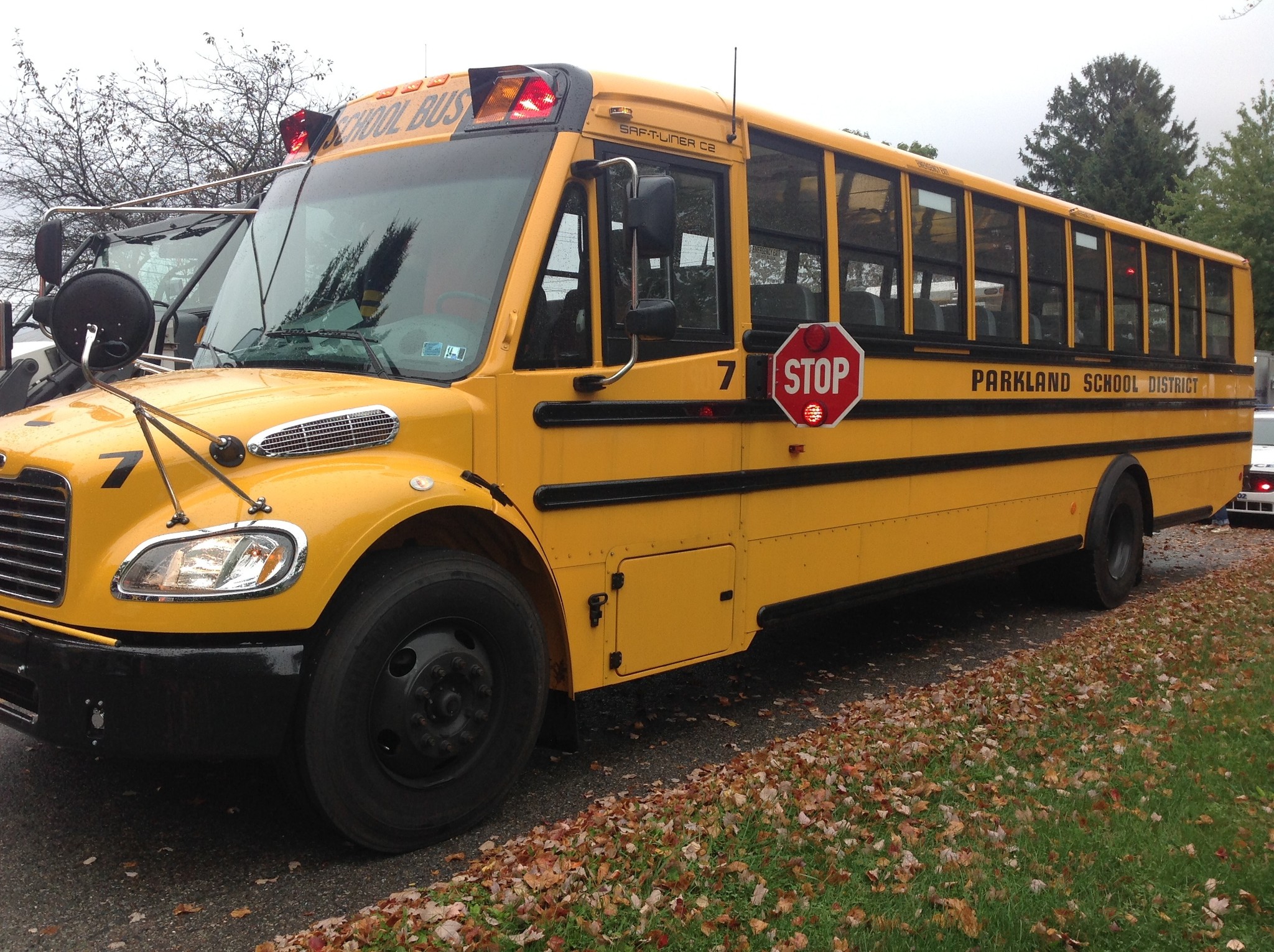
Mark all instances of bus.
[0,63,1254,854]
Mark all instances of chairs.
[574,227,1228,356]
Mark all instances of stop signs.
[773,321,865,428]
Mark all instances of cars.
[1223,407,1274,515]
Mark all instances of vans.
[0,192,268,409]
